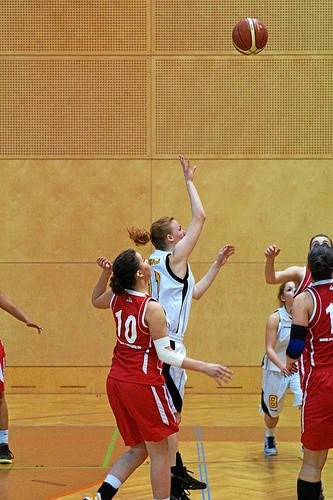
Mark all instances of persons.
[0,293,44,464]
[260,234,333,500]
[124,154,237,500]
[83,249,234,500]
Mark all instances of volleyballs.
[232,18,268,56]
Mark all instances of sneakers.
[264,435,278,455]
[82,492,102,500]
[170,450,207,490]
[301,445,304,451]
[170,476,190,500]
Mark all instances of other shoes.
[0,441,14,464]
